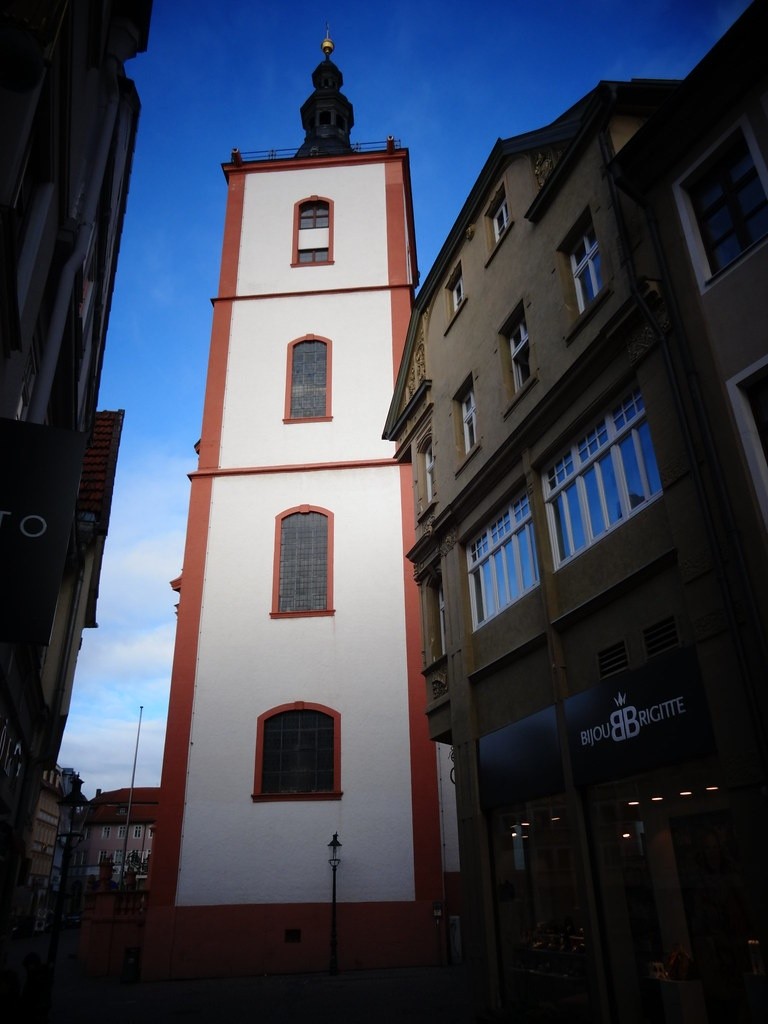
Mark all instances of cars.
[62,910,83,928]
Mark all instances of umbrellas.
[74,788,160,891]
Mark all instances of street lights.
[326,831,343,977]
[44,775,88,977]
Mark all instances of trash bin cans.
[69,917,81,930]
[120,946,140,984]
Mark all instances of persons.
[90,863,141,911]
[0,952,53,1024]
